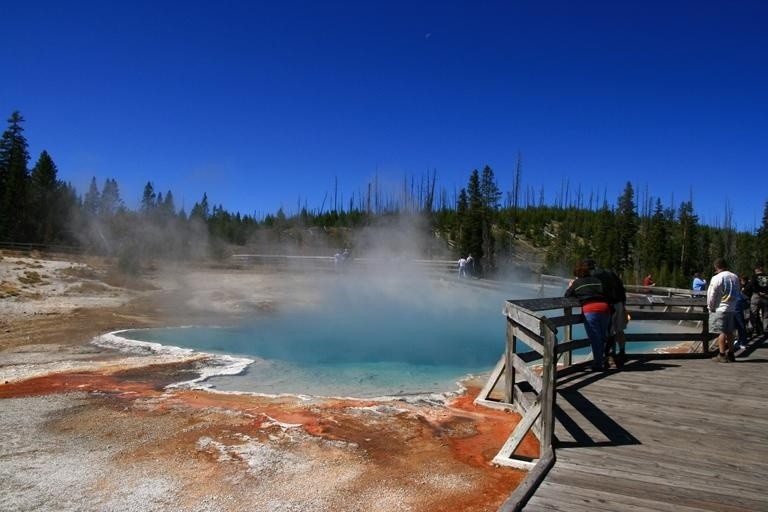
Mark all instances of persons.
[343,248,351,258]
[639,272,657,311]
[562,262,611,373]
[740,274,753,337]
[734,273,749,349]
[750,268,768,335]
[458,256,466,280]
[335,250,343,264]
[692,273,707,298]
[706,257,742,363]
[467,252,477,280]
[587,259,631,367]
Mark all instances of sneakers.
[712,340,747,363]
